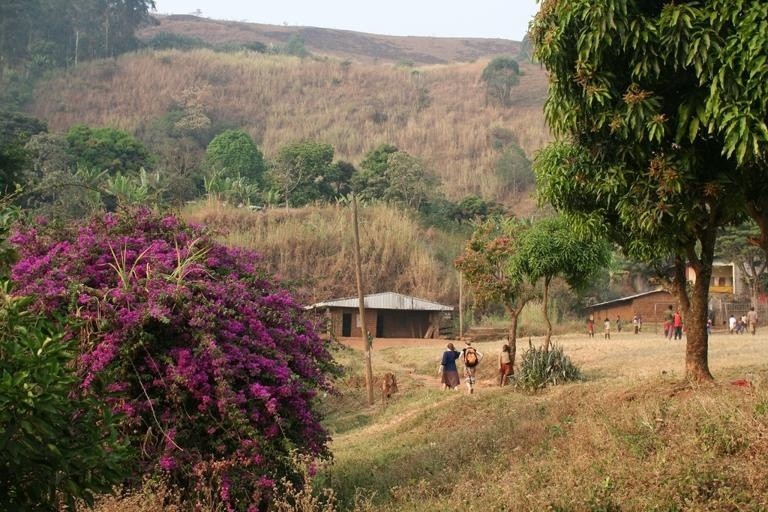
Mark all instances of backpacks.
[464,347,477,367]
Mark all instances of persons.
[459,341,484,394]
[617,315,621,332]
[498,345,511,387]
[728,306,758,335]
[707,317,714,334]
[604,318,611,340]
[664,303,683,340]
[633,312,642,334]
[438,343,460,392]
[587,314,594,337]
